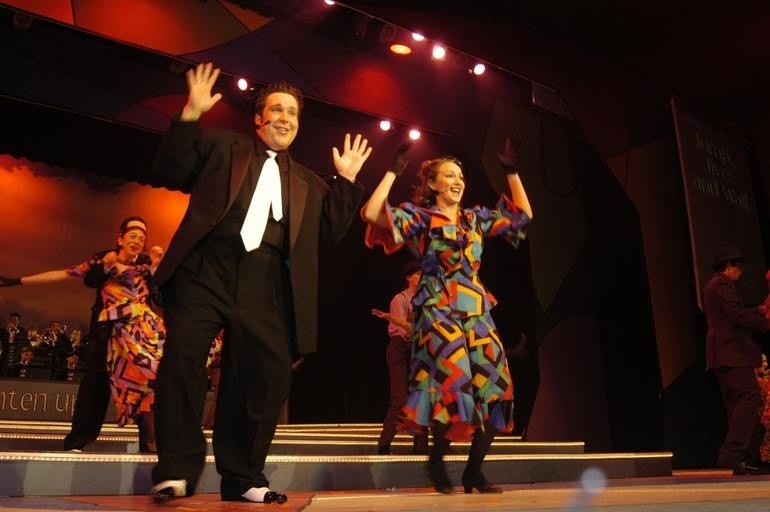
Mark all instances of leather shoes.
[151,479,186,501]
[720,460,758,473]
[240,488,288,504]
[139,432,156,451]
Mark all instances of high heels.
[428,460,452,493]
[462,467,502,493]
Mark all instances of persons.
[127,58,373,504]
[0,311,89,383]
[63,215,166,455]
[701,247,770,475]
[0,224,224,455]
[375,258,431,456]
[368,306,414,337]
[357,136,534,497]
[755,349,770,381]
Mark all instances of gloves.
[0,274,20,288]
[496,140,518,173]
[389,140,412,175]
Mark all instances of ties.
[240,150,282,252]
